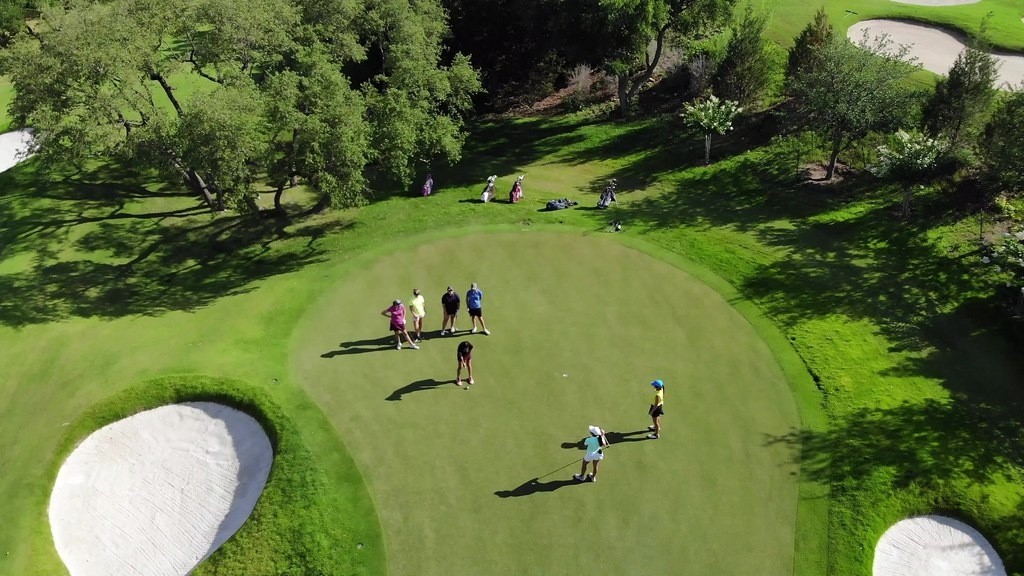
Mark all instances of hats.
[589,425,600,435]
[651,380,663,387]
[393,300,402,305]
[471,283,477,290]
[463,342,470,352]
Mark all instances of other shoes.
[650,426,660,430]
[575,474,585,481]
[472,328,477,333]
[484,329,490,334]
[410,344,419,349]
[397,343,401,349]
[441,330,445,335]
[589,472,596,481]
[457,379,462,385]
[468,377,474,384]
[648,434,659,438]
[451,327,455,332]
[415,337,421,343]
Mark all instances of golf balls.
[466,386,470,390]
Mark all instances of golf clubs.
[603,434,610,448]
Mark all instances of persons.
[381,300,419,349]
[421,173,433,197]
[575,426,610,482]
[409,288,427,343]
[441,286,461,335]
[457,341,474,386]
[646,379,665,439]
[466,283,490,335]
